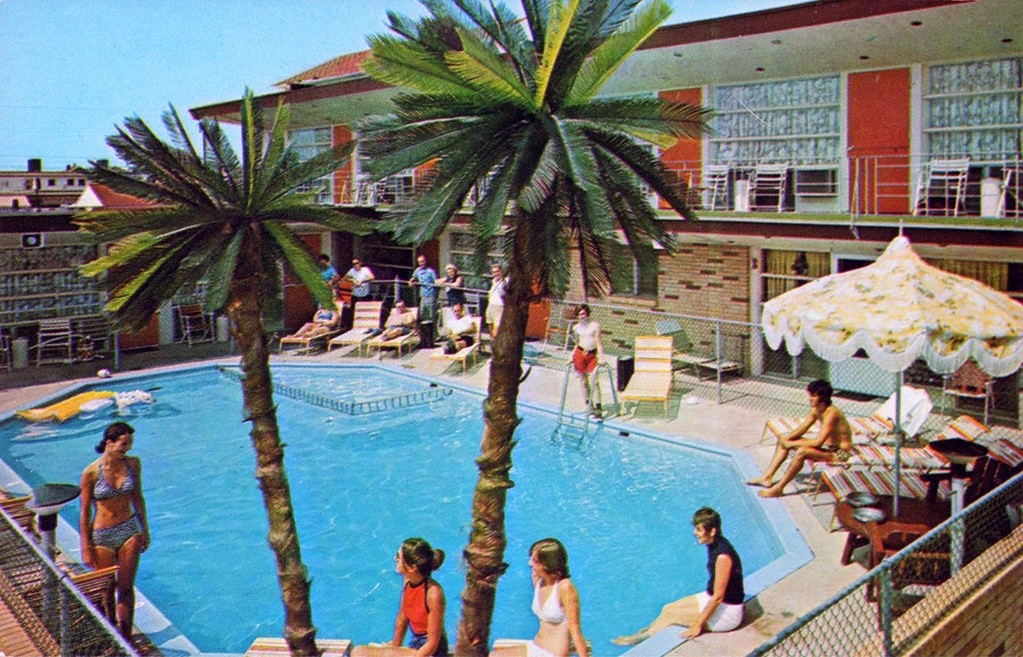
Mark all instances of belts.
[578,345,597,354]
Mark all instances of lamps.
[23,485,83,515]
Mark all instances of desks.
[837,491,951,601]
[828,357,901,398]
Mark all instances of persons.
[80,422,152,656]
[488,537,590,657]
[382,537,448,657]
[611,507,745,645]
[286,255,853,499]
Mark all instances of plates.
[852,507,886,523]
[845,491,880,506]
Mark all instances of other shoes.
[443,339,458,354]
[591,413,596,418]
[595,413,604,423]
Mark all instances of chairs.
[278,299,347,356]
[367,306,419,358]
[654,321,745,382]
[327,301,384,355]
[428,315,481,376]
[683,163,792,212]
[941,358,996,429]
[43,562,120,652]
[758,387,1023,632]
[617,335,675,423]
[914,157,971,219]
[544,304,577,354]
[1,489,36,536]
[35,319,75,369]
[174,301,214,348]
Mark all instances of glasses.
[353,262,359,266]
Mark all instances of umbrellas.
[761,225,1023,516]
